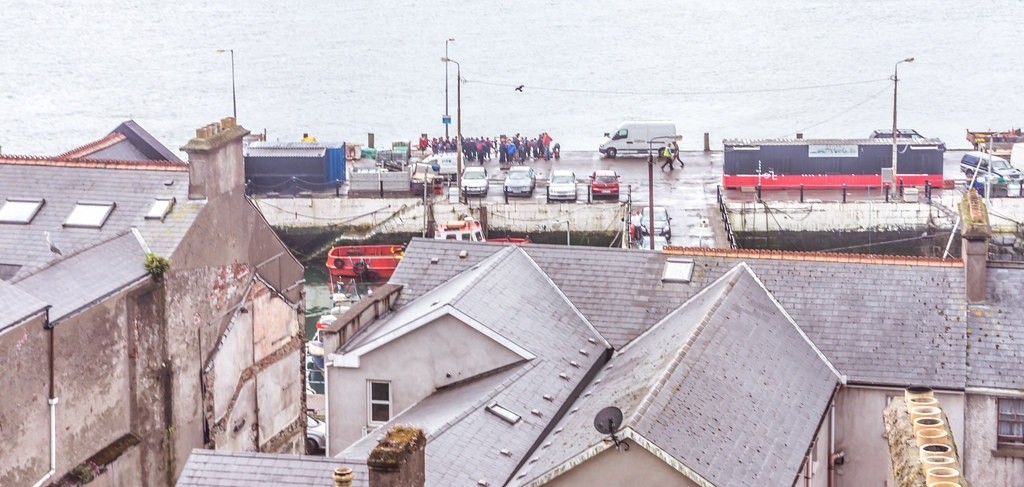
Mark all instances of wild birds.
[603,131,611,138]
[41,229,63,259]
[514,84,525,93]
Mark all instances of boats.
[305,275,372,395]
[325,218,527,279]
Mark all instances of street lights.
[216,48,238,122]
[648,132,684,250]
[439,57,463,202]
[891,56,915,199]
[444,38,455,138]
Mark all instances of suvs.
[960,150,1023,185]
[868,129,947,152]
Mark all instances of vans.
[598,120,678,158]
[422,151,465,180]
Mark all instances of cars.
[306,415,327,455]
[461,166,493,197]
[411,163,443,194]
[503,166,537,197]
[589,170,621,199]
[547,169,579,201]
[641,206,672,240]
[639,235,671,250]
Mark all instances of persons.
[418,133,561,165]
[631,211,644,240]
[661,140,683,171]
